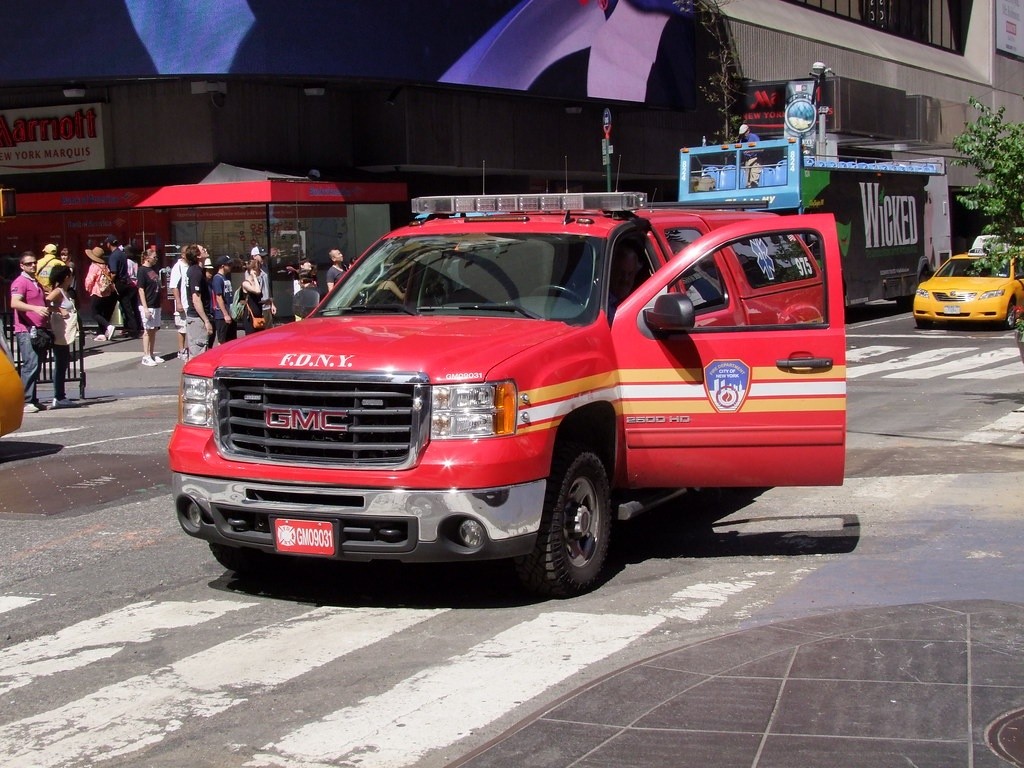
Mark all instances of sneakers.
[151,355,165,363]
[105,324,116,340]
[52,397,77,408]
[94,335,107,341]
[142,356,158,367]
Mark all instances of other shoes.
[178,348,188,361]
[23,402,47,412]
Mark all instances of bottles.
[30,326,37,339]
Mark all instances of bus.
[677,134,952,320]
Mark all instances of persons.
[137,249,164,367]
[34,244,77,296]
[170,244,278,361]
[46,264,80,406]
[85,236,140,341]
[292,260,317,322]
[10,254,52,413]
[326,249,358,292]
[368,259,407,306]
[739,124,764,188]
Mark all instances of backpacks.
[229,280,252,321]
[93,263,115,297]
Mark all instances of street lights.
[812,61,828,162]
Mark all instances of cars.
[913,235,1024,330]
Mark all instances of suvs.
[168,190,850,600]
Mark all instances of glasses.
[23,261,37,267]
[148,256,157,258]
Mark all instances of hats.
[104,235,118,246]
[739,124,748,134]
[85,247,106,264]
[250,246,269,257]
[216,255,233,265]
[42,244,58,254]
[203,258,214,268]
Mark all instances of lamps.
[63,88,86,98]
[304,88,325,95]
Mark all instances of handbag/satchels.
[30,327,55,352]
[253,318,266,329]
[68,287,77,299]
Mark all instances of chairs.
[701,158,935,191]
[448,251,520,302]
